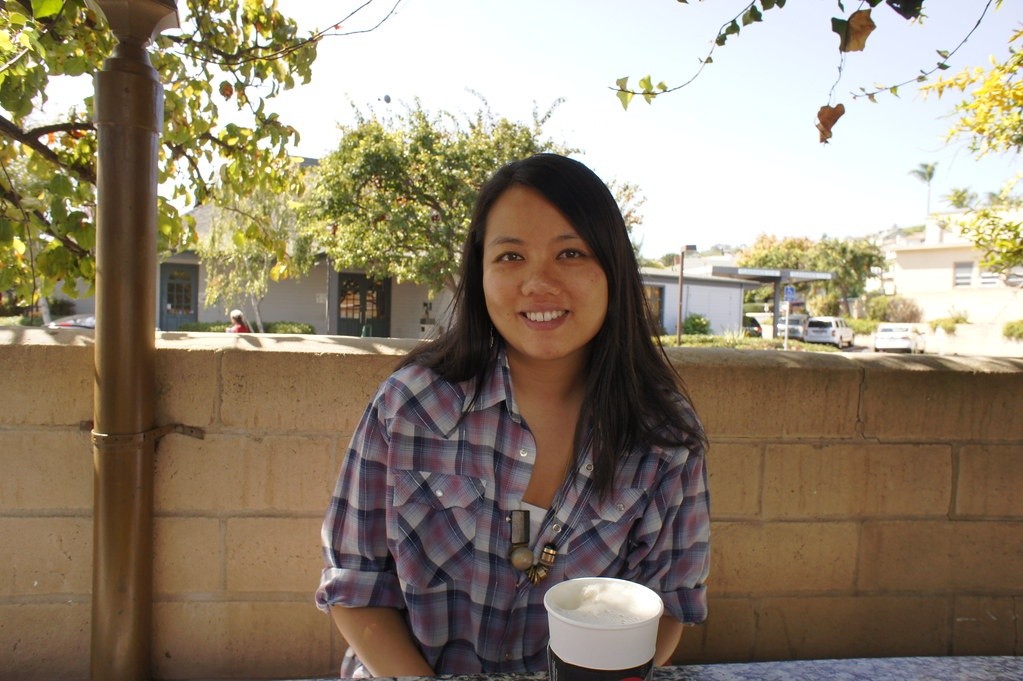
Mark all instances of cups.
[543,577,666,681]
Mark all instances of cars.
[42,314,96,330]
[803,316,855,349]
[742,313,773,338]
[875,324,927,354]
[777,314,809,339]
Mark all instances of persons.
[315,154,711,680]
[230,310,250,333]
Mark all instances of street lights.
[677,245,696,346]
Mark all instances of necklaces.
[510,433,576,586]
